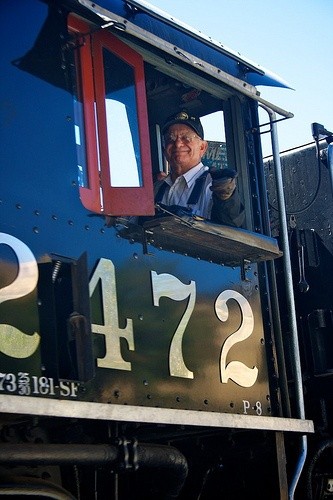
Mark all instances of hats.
[162,111,203,139]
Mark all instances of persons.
[153,109,247,229]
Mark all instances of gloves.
[207,168,238,200]
